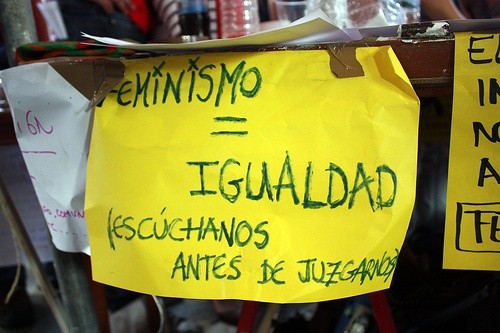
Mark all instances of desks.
[0,30,499,333]
